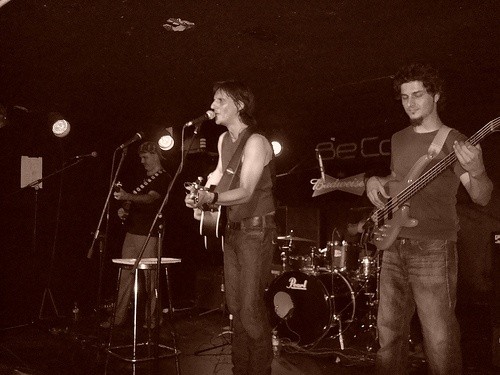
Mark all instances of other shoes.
[100,321,112,327]
[143,316,163,328]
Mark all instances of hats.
[138,142,167,160]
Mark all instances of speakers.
[274,206,320,266]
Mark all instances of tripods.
[199,273,231,316]
[194,315,233,354]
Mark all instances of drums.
[351,255,377,282]
[292,253,326,271]
[325,241,360,273]
[264,267,356,351]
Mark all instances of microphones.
[75,152,96,158]
[118,130,148,150]
[186,110,216,127]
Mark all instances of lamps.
[48,111,71,137]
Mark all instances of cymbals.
[277,235,315,242]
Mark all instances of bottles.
[72,301,80,322]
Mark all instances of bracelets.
[211,192,218,205]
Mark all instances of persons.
[186,78,279,375]
[98,141,171,336]
[366,64,493,375]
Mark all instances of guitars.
[362,116,500,250]
[115,180,131,225]
[190,177,225,253]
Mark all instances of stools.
[105,258,181,375]
